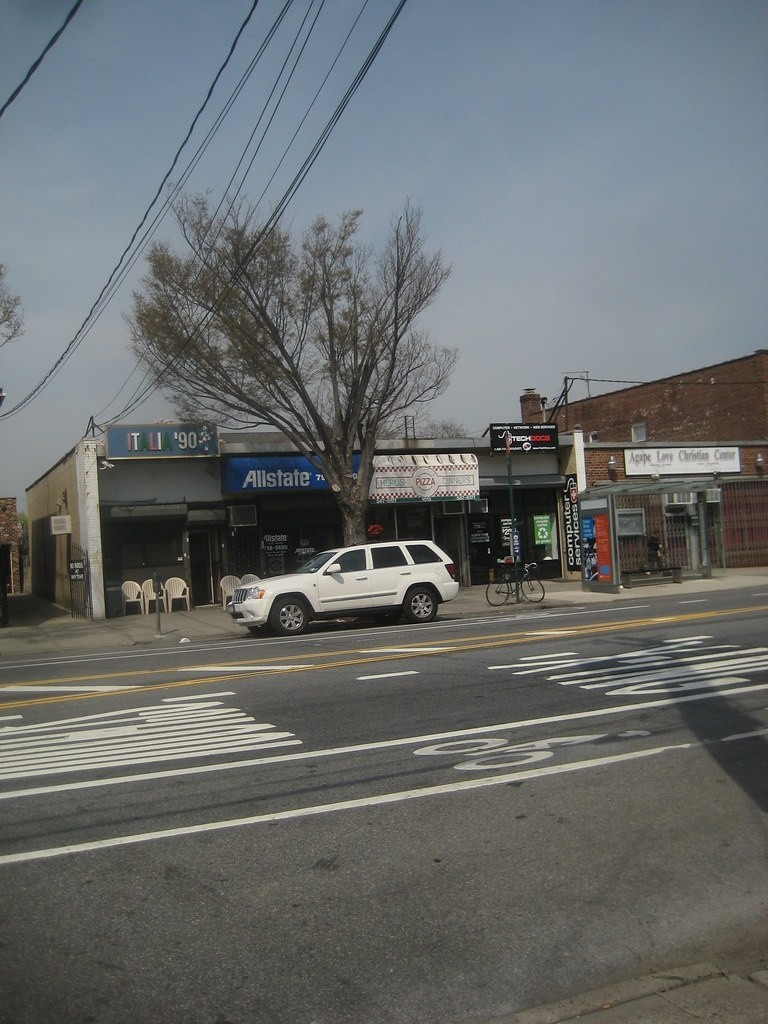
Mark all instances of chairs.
[120,580,144,616]
[141,579,167,615]
[241,574,260,585]
[219,575,241,612]
[164,577,191,613]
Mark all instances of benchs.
[622,566,682,589]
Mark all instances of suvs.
[226,538,459,637]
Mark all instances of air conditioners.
[226,504,257,527]
[663,493,693,506]
[468,498,489,514]
[439,500,465,516]
[48,515,72,536]
[696,489,721,503]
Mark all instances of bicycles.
[485,562,545,606]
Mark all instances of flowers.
[367,524,384,534]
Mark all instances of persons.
[645,529,666,574]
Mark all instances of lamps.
[713,471,719,479]
[754,453,764,466]
[99,459,115,472]
[651,473,661,481]
[56,497,62,507]
[608,456,617,470]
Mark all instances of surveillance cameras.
[99,459,115,468]
